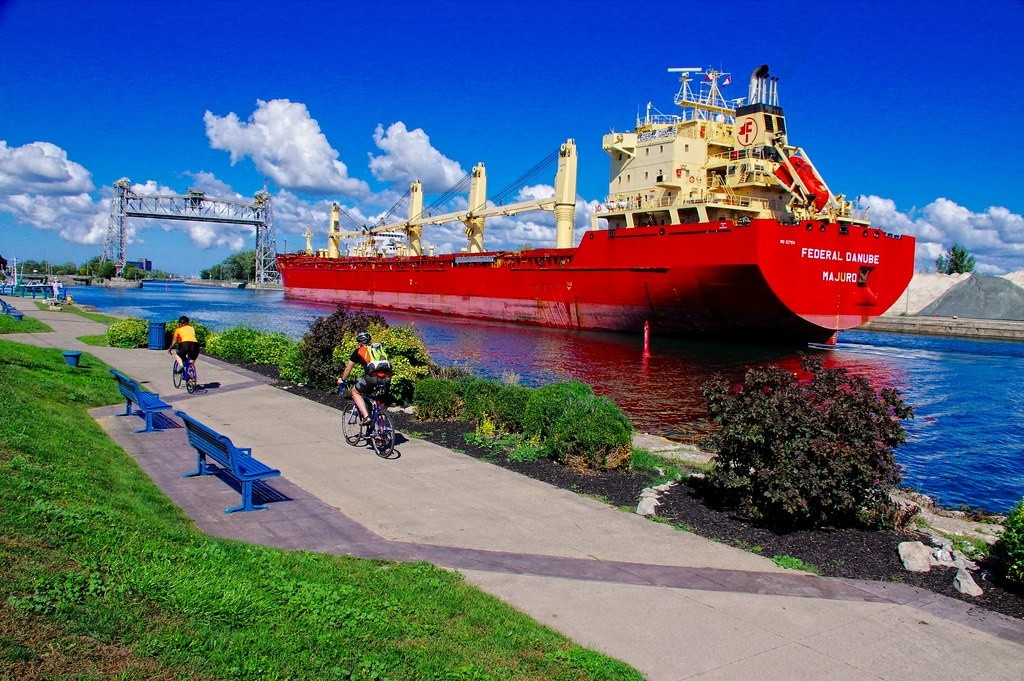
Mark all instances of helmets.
[178,316,189,323]
[356,332,371,342]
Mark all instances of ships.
[275,66,916,345]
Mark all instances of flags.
[705,72,713,81]
[722,75,732,88]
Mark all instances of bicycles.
[168,346,197,394]
[330,374,396,458]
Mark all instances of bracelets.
[341,377,347,381]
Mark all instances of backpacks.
[363,343,392,375]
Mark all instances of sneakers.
[359,416,371,426]
[177,365,184,373]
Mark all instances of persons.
[53,280,59,300]
[168,315,200,385]
[337,332,393,436]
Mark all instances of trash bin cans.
[147,320,166,350]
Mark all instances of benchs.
[110,369,172,433]
[0,299,16,315]
[175,410,280,514]
